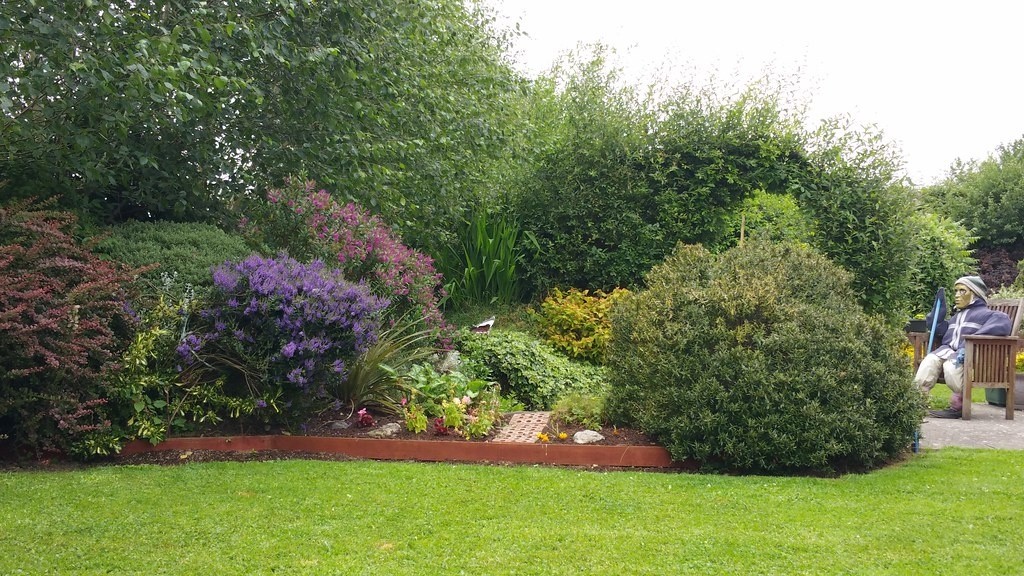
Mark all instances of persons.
[911,276,1011,419]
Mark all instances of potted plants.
[909,313,928,332]
[1007,348,1024,409]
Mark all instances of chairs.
[905,296,1024,421]
